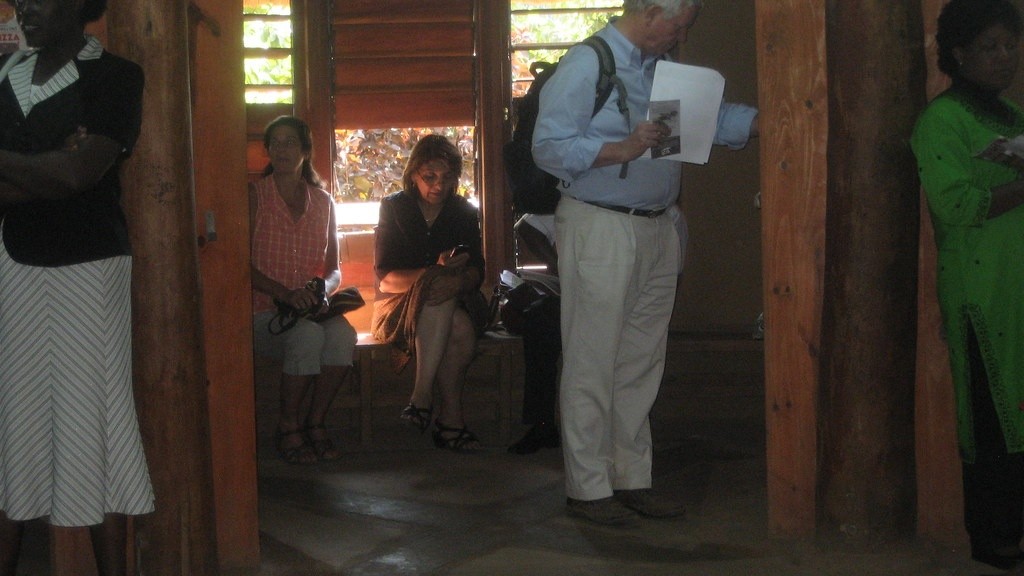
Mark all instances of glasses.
[416,169,454,183]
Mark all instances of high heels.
[400,403,432,436]
[432,414,482,451]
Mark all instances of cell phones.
[450,244,470,258]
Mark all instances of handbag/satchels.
[479,270,539,335]
[269,276,365,334]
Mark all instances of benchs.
[666,331,764,356]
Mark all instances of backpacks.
[502,36,630,215]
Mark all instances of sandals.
[304,421,342,461]
[274,423,319,466]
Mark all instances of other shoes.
[970,543,1024,570]
[508,422,559,454]
[613,489,685,518]
[567,496,641,528]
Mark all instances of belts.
[584,201,664,219]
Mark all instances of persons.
[506,0,762,525]
[240,116,359,465]
[0,0,160,576]
[370,133,494,453]
[907,0,1024,571]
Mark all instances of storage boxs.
[498,328,535,450]
[251,330,372,462]
[360,328,510,450]
